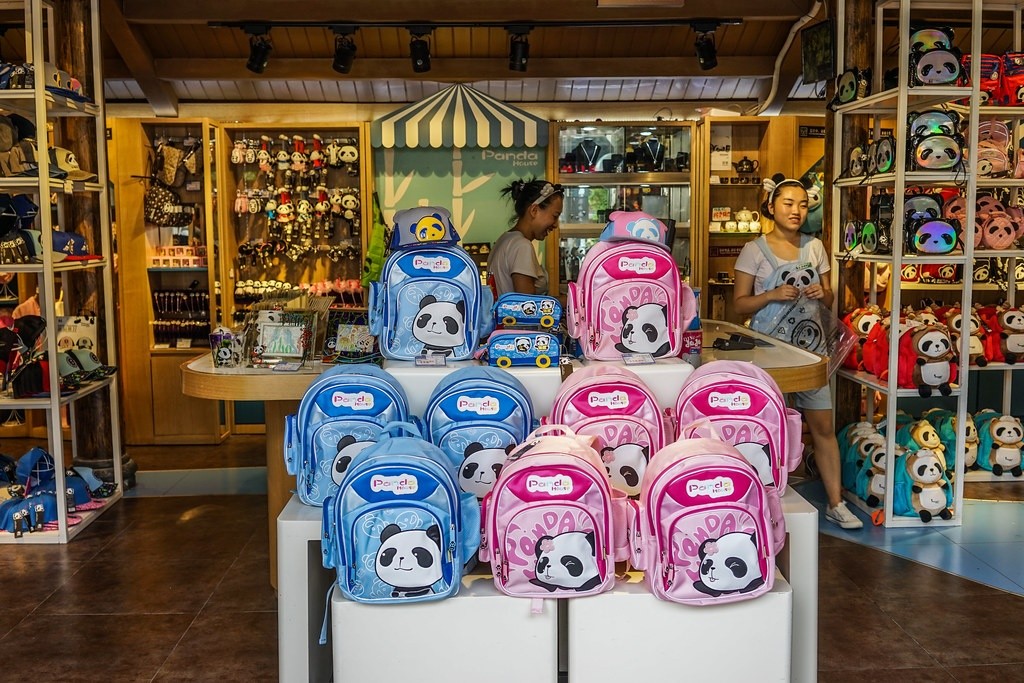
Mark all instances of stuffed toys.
[851,303,1024,523]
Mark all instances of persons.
[732,181,863,528]
[486,178,565,329]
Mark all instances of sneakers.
[826,500,863,528]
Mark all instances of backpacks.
[748,236,828,398]
[837,408,1024,526]
[829,110,1024,188]
[825,21,1023,107]
[843,194,1023,284]
[839,297,1022,400]
[282,240,804,645]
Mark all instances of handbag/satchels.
[143,140,214,227]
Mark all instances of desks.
[179,319,828,600]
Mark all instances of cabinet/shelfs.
[0,0,1024,683]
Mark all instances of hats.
[65,349,117,377]
[0,62,92,102]
[0,193,105,263]
[58,336,75,350]
[12,360,77,398]
[601,210,671,251]
[1,313,48,349]
[1,447,118,538]
[388,206,461,247]
[57,352,99,385]
[0,114,97,182]
[76,337,94,350]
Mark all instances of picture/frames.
[245,309,320,370]
[321,309,384,368]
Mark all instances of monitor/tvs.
[800,17,836,85]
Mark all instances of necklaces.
[647,143,660,164]
[581,144,598,166]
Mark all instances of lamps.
[404,24,437,74]
[505,26,534,72]
[691,24,722,71]
[240,25,272,75]
[328,27,360,75]
